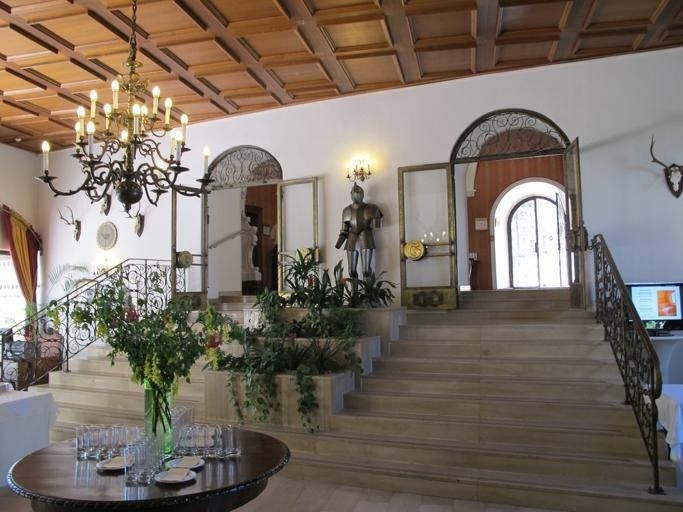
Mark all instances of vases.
[143,376,174,454]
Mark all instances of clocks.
[96,222,117,249]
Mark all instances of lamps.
[33,1,216,212]
[345,158,372,182]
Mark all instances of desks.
[637,333,683,384]
[642,385,682,465]
[0,389,53,487]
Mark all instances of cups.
[123,486,137,501]
[201,460,239,488]
[137,486,149,502]
[74,406,244,461]
[72,461,102,488]
[123,461,162,486]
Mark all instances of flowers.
[46,263,234,437]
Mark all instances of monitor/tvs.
[621,282,682,336]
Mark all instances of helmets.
[350,182,365,202]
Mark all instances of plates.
[154,469,197,485]
[97,457,135,471]
[165,456,206,470]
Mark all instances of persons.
[331,179,386,303]
